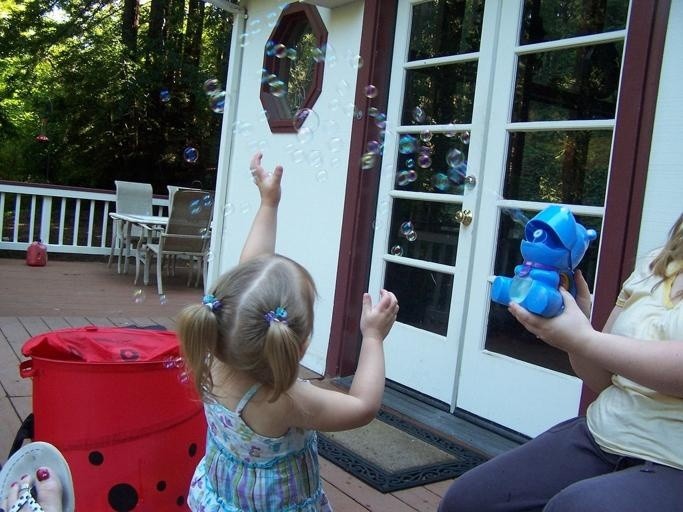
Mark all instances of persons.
[174,150,403,512]
[436,209,683,512]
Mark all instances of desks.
[108,211,212,285]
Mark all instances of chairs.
[166,183,203,283]
[106,179,153,273]
[140,188,214,297]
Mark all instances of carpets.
[315,408,491,494]
